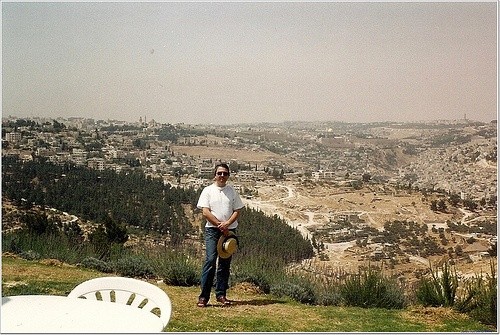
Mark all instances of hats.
[217,231,239,258]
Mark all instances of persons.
[194,163,246,308]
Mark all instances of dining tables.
[1,293,165,333]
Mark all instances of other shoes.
[217,296,233,305]
[197,297,208,306]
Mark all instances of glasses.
[216,172,229,176]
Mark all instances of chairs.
[64,276,173,332]
